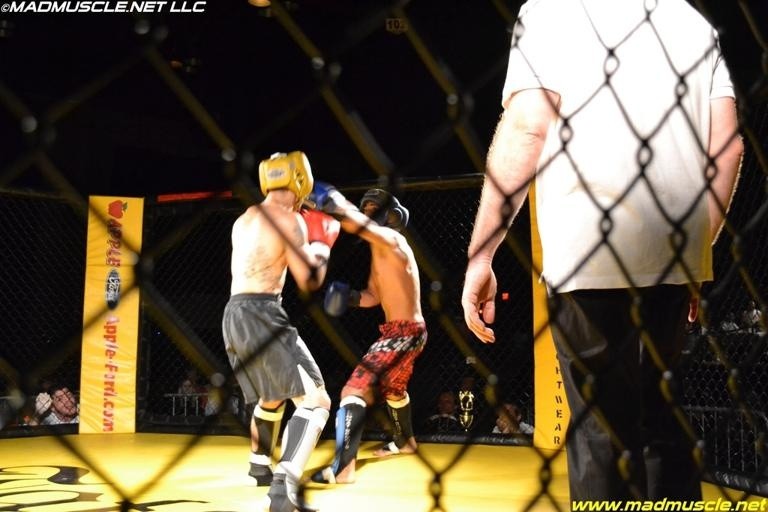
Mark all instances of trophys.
[458,390,474,433]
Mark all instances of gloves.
[324,279,360,317]
[309,182,347,220]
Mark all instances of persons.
[303,182,428,484]
[221,150,340,512]
[460,0,745,512]
[720,312,739,331]
[423,391,479,434]
[741,300,762,325]
[41,383,80,425]
[491,403,534,434]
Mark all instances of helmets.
[359,188,411,233]
[259,151,314,211]
